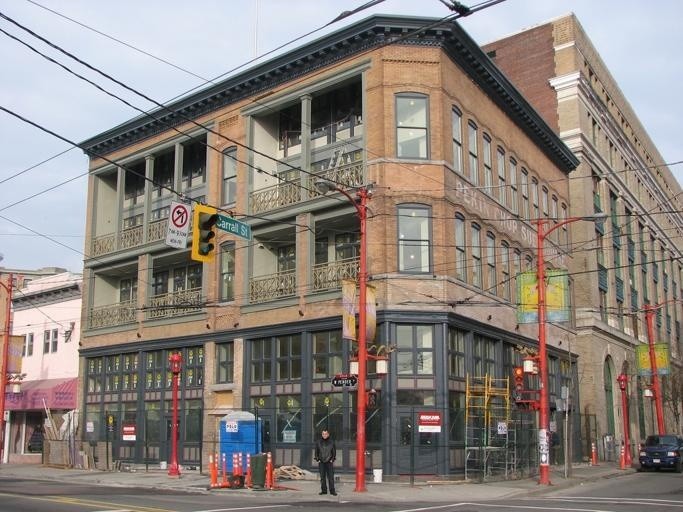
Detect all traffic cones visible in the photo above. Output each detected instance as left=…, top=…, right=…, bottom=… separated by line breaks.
left=208, top=450, right=275, bottom=491
left=620, top=445, right=627, bottom=470
left=588, top=440, right=597, bottom=465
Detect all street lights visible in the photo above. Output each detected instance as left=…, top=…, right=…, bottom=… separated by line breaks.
left=170, top=353, right=182, bottom=476
left=641, top=300, right=683, bottom=434
left=535, top=214, right=608, bottom=485
left=617, top=375, right=634, bottom=469
left=314, top=179, right=368, bottom=491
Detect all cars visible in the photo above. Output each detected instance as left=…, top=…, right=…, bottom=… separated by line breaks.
left=639, top=434, right=682, bottom=468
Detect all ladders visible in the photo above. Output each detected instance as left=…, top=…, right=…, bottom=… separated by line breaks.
left=465, top=372, right=516, bottom=483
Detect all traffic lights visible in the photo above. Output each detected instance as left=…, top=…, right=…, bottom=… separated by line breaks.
left=191, top=206, right=219, bottom=264
left=514, top=367, right=525, bottom=391
left=106, top=413, right=115, bottom=429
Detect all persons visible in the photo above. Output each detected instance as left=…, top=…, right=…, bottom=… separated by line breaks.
left=314, top=429, right=338, bottom=496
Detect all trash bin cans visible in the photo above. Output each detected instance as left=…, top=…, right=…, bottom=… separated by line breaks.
left=251, top=453, right=266, bottom=489
